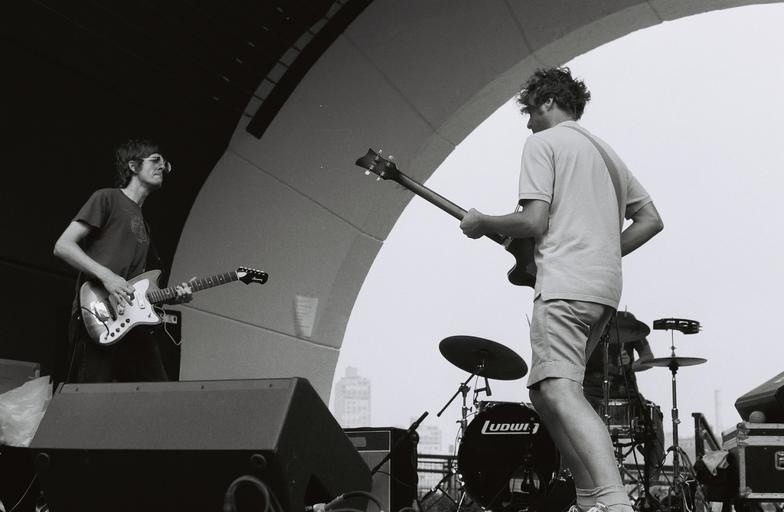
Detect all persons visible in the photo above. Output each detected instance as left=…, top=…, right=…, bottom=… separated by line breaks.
left=585, top=309, right=666, bottom=498
left=48, top=134, right=196, bottom=512
left=458, top=62, right=667, bottom=512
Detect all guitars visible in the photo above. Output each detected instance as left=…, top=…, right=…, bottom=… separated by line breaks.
left=354, top=148, right=537, bottom=290
left=80, top=266, right=269, bottom=346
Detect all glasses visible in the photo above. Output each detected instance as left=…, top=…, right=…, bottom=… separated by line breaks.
left=139, top=156, right=172, bottom=174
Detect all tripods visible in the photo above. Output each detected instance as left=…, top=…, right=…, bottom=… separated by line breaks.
left=639, top=369, right=717, bottom=512
left=419, top=387, right=483, bottom=511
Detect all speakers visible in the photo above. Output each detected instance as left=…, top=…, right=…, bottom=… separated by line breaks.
left=342, top=426, right=416, bottom=512
left=734, top=369, right=783, bottom=423
left=29, top=375, right=373, bottom=512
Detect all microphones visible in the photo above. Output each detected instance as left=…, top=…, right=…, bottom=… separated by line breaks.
left=520, top=455, right=534, bottom=492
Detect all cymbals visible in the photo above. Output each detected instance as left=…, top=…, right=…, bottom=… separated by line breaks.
left=640, top=357, right=706, bottom=368
left=603, top=314, right=650, bottom=344
left=440, top=337, right=528, bottom=380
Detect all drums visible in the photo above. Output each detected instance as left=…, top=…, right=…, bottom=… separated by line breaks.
left=589, top=399, right=662, bottom=439
left=455, top=400, right=577, bottom=509
